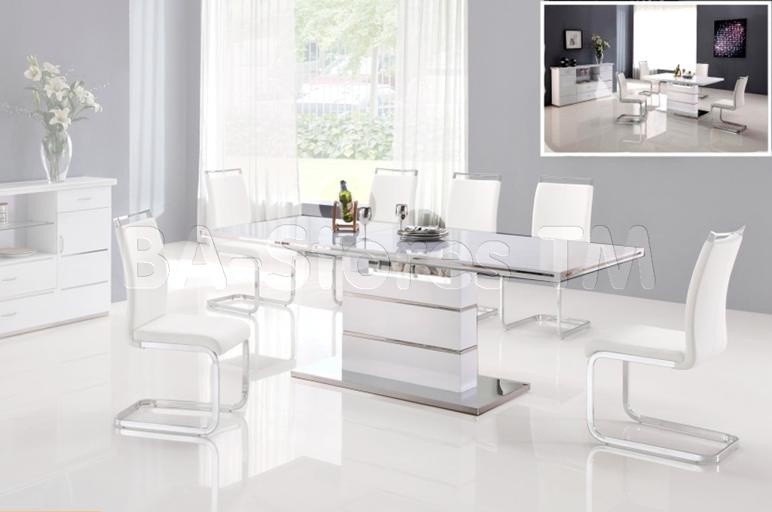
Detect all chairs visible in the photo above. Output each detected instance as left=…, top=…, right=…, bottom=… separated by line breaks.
left=113, top=209, right=250, bottom=437
left=586, top=226, right=749, bottom=463
left=200, top=166, right=296, bottom=315
left=365, top=168, right=418, bottom=222
left=446, top=173, right=501, bottom=233
left=497, top=182, right=594, bottom=339
left=617, top=60, right=749, bottom=135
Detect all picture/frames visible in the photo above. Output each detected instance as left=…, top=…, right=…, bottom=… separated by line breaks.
left=563, top=26, right=584, bottom=51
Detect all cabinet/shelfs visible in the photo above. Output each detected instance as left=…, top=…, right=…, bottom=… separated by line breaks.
left=1, top=176, right=120, bottom=341
left=549, top=62, right=615, bottom=108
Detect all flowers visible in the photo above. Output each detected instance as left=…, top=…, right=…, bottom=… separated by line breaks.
left=591, top=33, right=612, bottom=62
left=1, top=49, right=111, bottom=181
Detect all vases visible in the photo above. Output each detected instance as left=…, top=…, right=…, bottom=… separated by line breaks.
left=594, top=52, right=604, bottom=65
left=36, top=128, right=76, bottom=180
